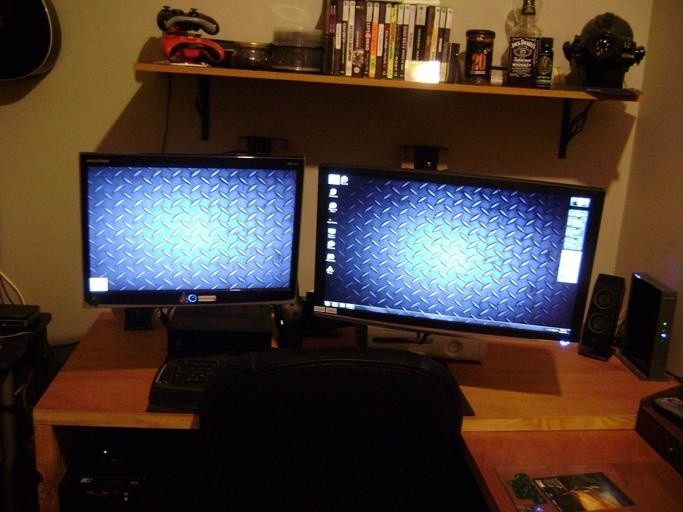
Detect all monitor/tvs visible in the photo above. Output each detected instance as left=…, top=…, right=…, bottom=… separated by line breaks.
left=312, top=166, right=605, bottom=363
left=81, top=153, right=306, bottom=350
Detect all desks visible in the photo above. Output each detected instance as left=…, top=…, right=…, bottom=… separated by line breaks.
left=31, top=312, right=683, bottom=511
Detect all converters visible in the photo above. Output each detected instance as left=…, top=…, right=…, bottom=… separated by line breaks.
left=279, top=321, right=302, bottom=350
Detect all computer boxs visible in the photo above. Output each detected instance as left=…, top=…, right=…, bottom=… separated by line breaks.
left=59, top=449, right=146, bottom=512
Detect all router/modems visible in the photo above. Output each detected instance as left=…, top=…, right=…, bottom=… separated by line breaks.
left=0, top=304, right=40, bottom=328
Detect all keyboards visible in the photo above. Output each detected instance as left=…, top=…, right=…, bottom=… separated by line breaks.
left=149, top=357, right=229, bottom=413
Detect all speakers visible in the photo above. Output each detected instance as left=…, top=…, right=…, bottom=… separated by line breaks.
left=578, top=273, right=626, bottom=362
left=123, top=308, right=154, bottom=330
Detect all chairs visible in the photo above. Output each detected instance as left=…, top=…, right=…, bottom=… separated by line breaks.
left=196, top=345, right=469, bottom=512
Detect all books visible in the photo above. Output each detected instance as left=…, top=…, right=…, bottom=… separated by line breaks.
left=323, top=1, right=460, bottom=84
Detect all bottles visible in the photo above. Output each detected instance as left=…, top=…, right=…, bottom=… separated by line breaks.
left=505, top=0, right=541, bottom=87
left=535, top=36, right=554, bottom=89
